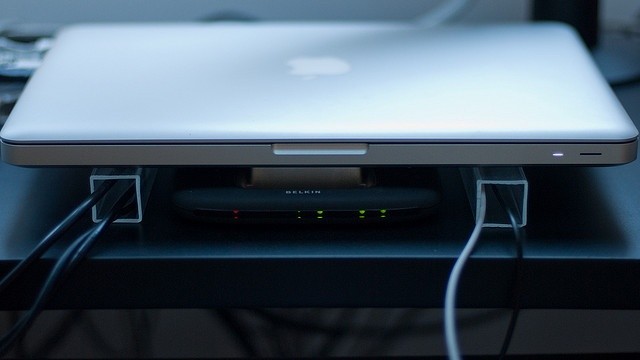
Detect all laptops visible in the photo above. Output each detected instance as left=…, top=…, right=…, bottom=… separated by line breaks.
left=1, top=19, right=639, bottom=168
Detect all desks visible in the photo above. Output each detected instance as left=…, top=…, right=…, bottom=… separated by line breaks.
left=1, top=30, right=640, bottom=359
